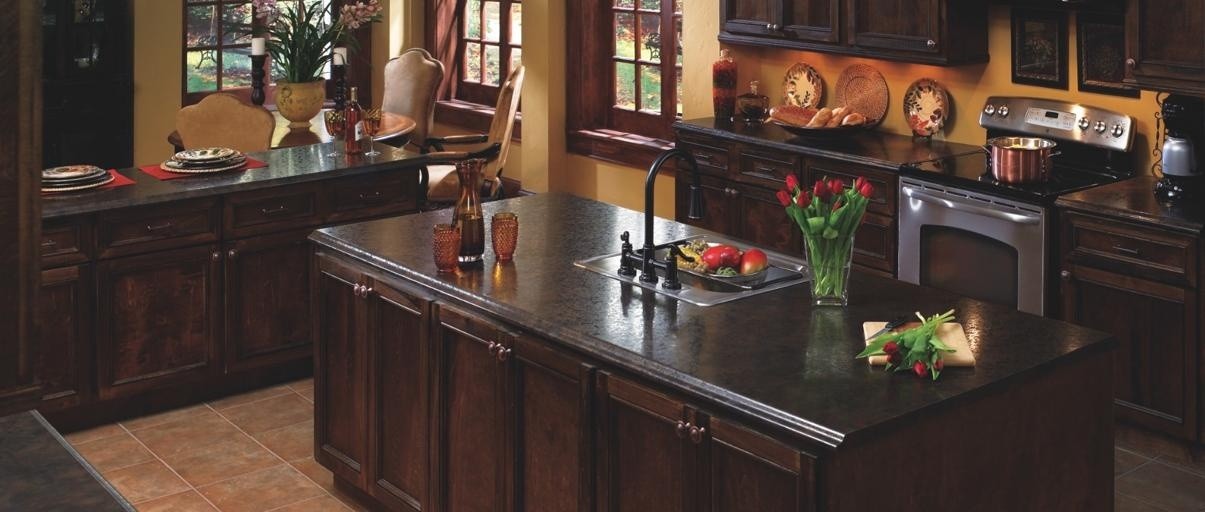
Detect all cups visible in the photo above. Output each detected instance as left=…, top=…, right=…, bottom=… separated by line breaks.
left=325, top=110, right=343, bottom=157
left=433, top=224, right=461, bottom=272
left=492, top=213, right=518, bottom=263
left=802, top=234, right=854, bottom=306
left=361, top=109, right=381, bottom=156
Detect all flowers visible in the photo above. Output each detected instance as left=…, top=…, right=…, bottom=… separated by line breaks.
left=776, top=175, right=873, bottom=297
left=223, top=0, right=383, bottom=83
left=854, top=308, right=957, bottom=382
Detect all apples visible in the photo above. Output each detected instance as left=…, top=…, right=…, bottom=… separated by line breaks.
left=739, top=249, right=768, bottom=275
left=700, top=244, right=743, bottom=269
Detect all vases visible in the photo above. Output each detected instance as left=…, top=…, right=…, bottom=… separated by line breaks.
left=274, top=77, right=327, bottom=127
left=802, top=234, right=854, bottom=308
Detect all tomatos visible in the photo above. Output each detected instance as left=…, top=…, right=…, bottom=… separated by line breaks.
left=741, top=248, right=768, bottom=275
left=701, top=244, right=741, bottom=270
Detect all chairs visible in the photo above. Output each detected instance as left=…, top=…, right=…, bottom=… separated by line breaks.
left=175, top=92, right=276, bottom=151
left=417, top=65, right=526, bottom=212
left=379, top=47, right=445, bottom=144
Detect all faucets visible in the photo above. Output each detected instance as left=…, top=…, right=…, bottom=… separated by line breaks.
left=618, top=149, right=705, bottom=290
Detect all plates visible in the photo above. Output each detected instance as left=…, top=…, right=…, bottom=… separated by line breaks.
left=159, top=160, right=246, bottom=173
left=773, top=119, right=861, bottom=139
left=834, top=64, right=888, bottom=126
left=783, top=63, right=822, bottom=109
left=707, top=265, right=771, bottom=287
left=41, top=175, right=115, bottom=192
left=903, top=78, right=950, bottom=137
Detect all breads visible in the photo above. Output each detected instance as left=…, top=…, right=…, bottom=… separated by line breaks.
left=768, top=104, right=821, bottom=127
left=824, top=105, right=851, bottom=129
left=804, top=107, right=832, bottom=129
left=842, top=110, right=866, bottom=126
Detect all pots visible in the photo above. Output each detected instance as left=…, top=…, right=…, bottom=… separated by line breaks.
left=982, top=136, right=1061, bottom=184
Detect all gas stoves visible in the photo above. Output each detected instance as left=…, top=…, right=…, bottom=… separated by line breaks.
left=897, top=96, right=1139, bottom=317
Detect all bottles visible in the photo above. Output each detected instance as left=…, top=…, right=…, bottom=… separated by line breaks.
left=344, top=86, right=363, bottom=156
left=713, top=49, right=738, bottom=120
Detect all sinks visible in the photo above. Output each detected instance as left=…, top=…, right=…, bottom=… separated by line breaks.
left=635, top=234, right=807, bottom=295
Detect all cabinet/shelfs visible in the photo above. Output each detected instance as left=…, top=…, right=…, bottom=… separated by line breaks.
left=95, top=197, right=225, bottom=409
left=843, top=0, right=990, bottom=69
left=716, top=1, right=843, bottom=55
left=330, top=166, right=420, bottom=225
left=312, top=244, right=434, bottom=512
left=223, top=180, right=331, bottom=383
left=675, top=128, right=801, bottom=259
left=593, top=328, right=1118, bottom=511
left=801, top=151, right=897, bottom=278
left=1053, top=209, right=1202, bottom=445
left=28, top=212, right=94, bottom=418
left=429, top=299, right=597, bottom=512
